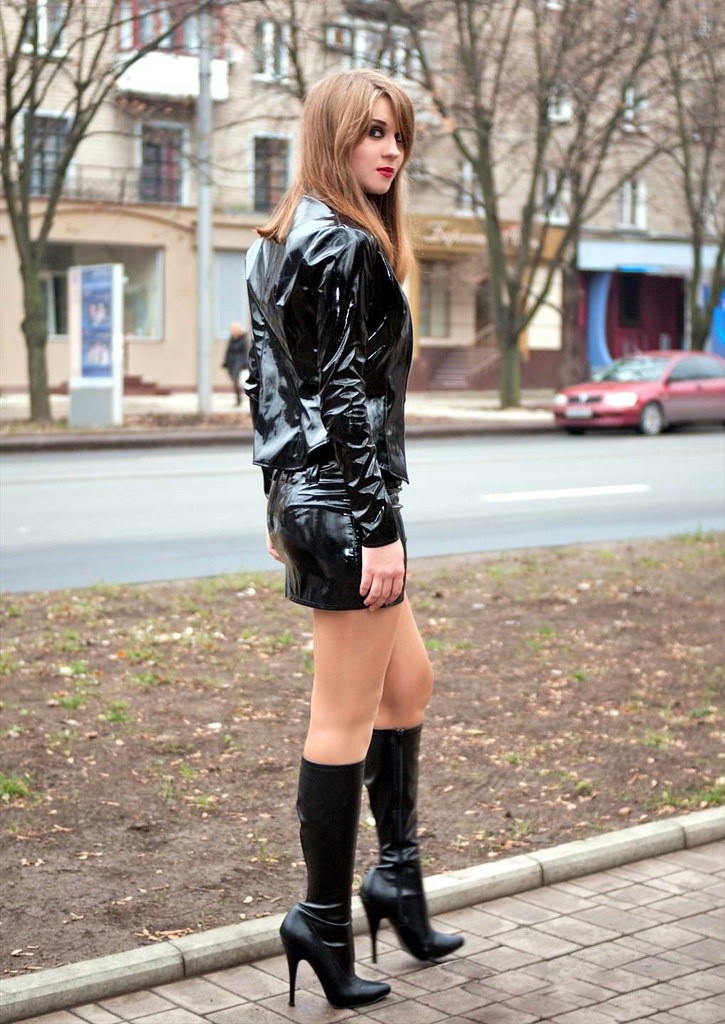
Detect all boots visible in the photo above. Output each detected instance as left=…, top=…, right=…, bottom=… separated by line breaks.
left=279, top=756, right=390, bottom=1008
left=363, top=724, right=464, bottom=961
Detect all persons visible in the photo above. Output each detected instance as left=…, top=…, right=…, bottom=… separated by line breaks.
left=222, top=323, right=249, bottom=406
left=244, top=67, right=466, bottom=1010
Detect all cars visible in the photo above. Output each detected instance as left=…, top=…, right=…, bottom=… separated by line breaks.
left=550, top=350, right=725, bottom=437
